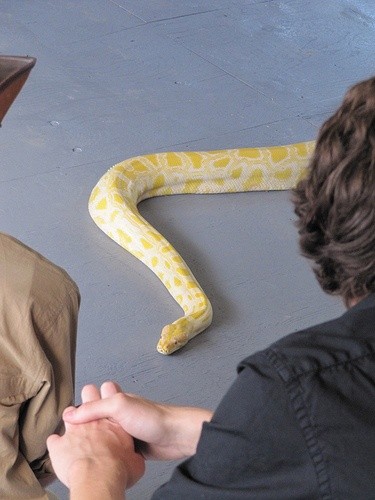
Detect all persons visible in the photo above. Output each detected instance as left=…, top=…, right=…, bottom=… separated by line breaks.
left=1, top=228, right=82, bottom=500
left=45, top=74, right=375, bottom=500
